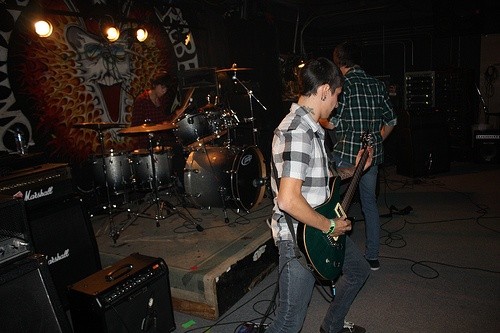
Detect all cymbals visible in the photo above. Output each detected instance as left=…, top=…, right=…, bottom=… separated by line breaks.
left=214, top=67, right=256, bottom=72
left=70, top=122, right=129, bottom=130
left=118, top=124, right=181, bottom=134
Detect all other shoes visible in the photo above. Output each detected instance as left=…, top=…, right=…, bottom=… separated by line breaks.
left=319, top=320, right=367, bottom=333
left=367, top=260, right=380, bottom=270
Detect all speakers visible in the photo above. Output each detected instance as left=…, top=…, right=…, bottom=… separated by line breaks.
left=397, top=106, right=475, bottom=178
left=0, top=150, right=176, bottom=333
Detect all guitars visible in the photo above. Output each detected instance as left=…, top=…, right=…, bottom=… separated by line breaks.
left=297, top=136, right=374, bottom=285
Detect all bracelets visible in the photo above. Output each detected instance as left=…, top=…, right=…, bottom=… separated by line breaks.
left=322, top=219, right=335, bottom=235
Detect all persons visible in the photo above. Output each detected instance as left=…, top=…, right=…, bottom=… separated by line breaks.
left=132, top=73, right=183, bottom=187
left=262, top=56, right=373, bottom=333
left=324, top=43, right=398, bottom=270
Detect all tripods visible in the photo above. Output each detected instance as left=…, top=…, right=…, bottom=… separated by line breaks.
left=88, top=120, right=242, bottom=244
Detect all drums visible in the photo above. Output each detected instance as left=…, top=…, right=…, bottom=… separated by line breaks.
left=88, top=103, right=268, bottom=213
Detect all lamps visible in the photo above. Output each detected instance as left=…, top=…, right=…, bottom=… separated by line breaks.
left=176, top=31, right=191, bottom=45
left=29, top=17, right=55, bottom=38
left=100, top=24, right=149, bottom=43
